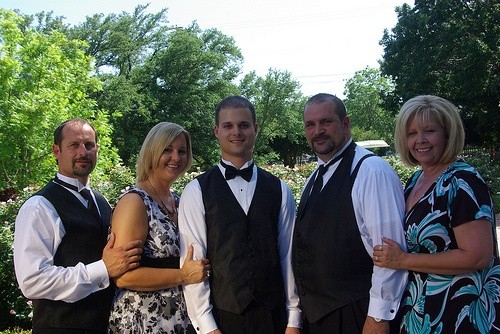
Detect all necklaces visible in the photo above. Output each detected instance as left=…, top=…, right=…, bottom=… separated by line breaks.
left=146, top=177, right=178, bottom=217
left=411, top=171, right=438, bottom=197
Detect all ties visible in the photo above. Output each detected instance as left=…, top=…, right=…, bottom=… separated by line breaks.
left=54, top=176, right=100, bottom=225
left=300, top=147, right=348, bottom=221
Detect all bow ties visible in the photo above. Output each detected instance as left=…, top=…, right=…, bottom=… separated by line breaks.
left=220, top=160, right=254, bottom=182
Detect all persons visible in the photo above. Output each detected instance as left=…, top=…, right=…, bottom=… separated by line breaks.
left=291, top=93, right=408, bottom=334
left=178, top=96, right=304, bottom=334
left=373, top=94, right=500, bottom=334
left=14, top=118, right=143, bottom=334
left=109, top=122, right=212, bottom=334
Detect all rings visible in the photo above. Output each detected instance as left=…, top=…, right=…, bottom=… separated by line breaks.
left=375, top=256, right=379, bottom=262
left=378, top=245, right=383, bottom=252
left=206, top=270, right=211, bottom=277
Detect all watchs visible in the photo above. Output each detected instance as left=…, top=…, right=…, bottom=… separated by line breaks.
left=371, top=316, right=385, bottom=323
